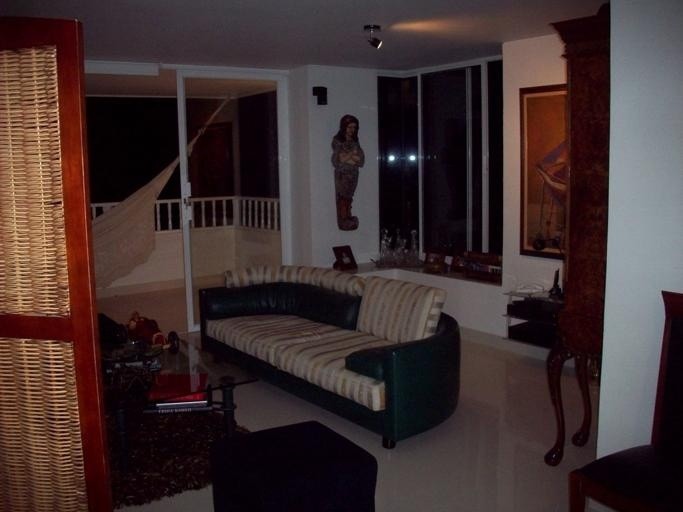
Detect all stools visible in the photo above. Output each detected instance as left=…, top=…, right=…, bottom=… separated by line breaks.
left=206, top=419, right=377, bottom=511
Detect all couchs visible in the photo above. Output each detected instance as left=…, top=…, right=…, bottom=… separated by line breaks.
left=197, top=265, right=461, bottom=451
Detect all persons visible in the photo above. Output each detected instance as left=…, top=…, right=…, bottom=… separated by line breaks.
left=330, top=113, right=366, bottom=231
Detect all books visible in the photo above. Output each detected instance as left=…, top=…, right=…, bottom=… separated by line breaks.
left=143, top=392, right=213, bottom=414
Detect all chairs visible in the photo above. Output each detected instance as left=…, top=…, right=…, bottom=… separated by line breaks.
left=565, top=291, right=682, bottom=512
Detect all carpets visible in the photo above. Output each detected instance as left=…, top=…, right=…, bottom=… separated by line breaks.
left=105, top=410, right=251, bottom=507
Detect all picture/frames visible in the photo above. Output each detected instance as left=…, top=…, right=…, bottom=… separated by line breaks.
left=517, top=84, right=568, bottom=259
left=332, top=245, right=357, bottom=272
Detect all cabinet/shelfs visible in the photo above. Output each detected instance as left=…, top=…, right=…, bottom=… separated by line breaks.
left=500, top=290, right=571, bottom=358
